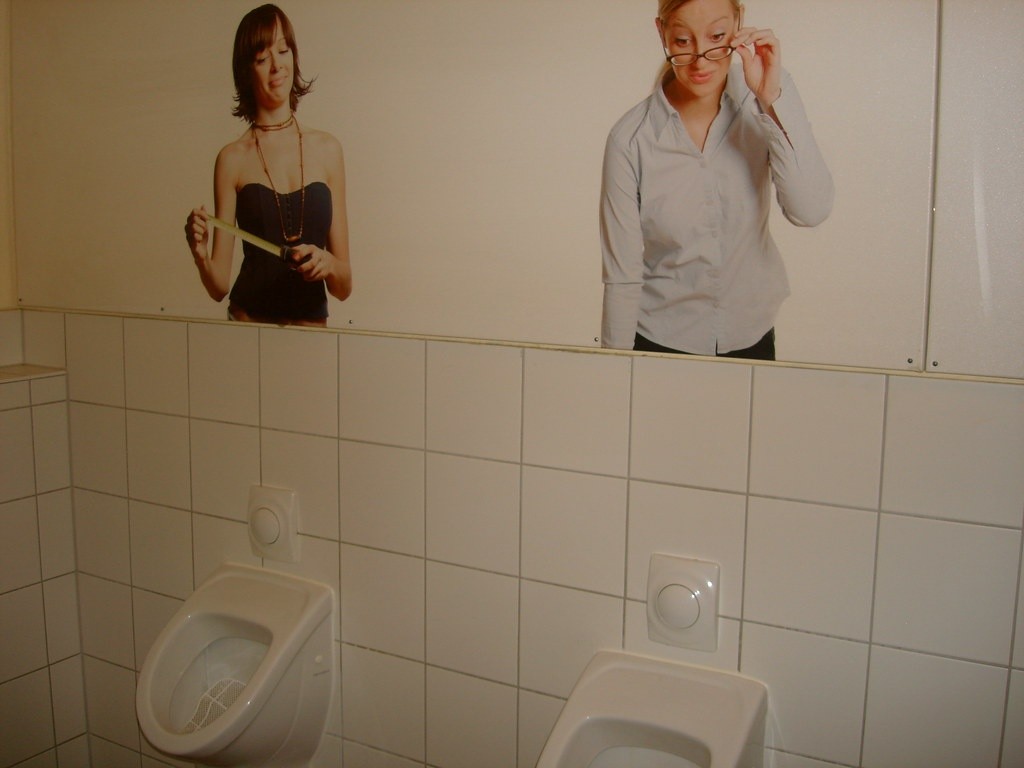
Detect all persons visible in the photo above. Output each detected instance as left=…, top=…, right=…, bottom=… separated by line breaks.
left=600, top=0, right=833, bottom=360
left=184, top=5, right=353, bottom=328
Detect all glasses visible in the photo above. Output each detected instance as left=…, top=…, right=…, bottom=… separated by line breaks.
left=661, top=8, right=740, bottom=66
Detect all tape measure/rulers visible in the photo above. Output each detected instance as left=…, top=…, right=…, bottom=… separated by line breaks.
left=205, top=214, right=311, bottom=266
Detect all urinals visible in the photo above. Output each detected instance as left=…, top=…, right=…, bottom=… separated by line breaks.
left=134, top=565, right=334, bottom=767
left=532, top=651, right=768, bottom=768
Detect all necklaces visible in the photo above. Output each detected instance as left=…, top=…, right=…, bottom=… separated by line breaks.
left=254, top=113, right=306, bottom=242
